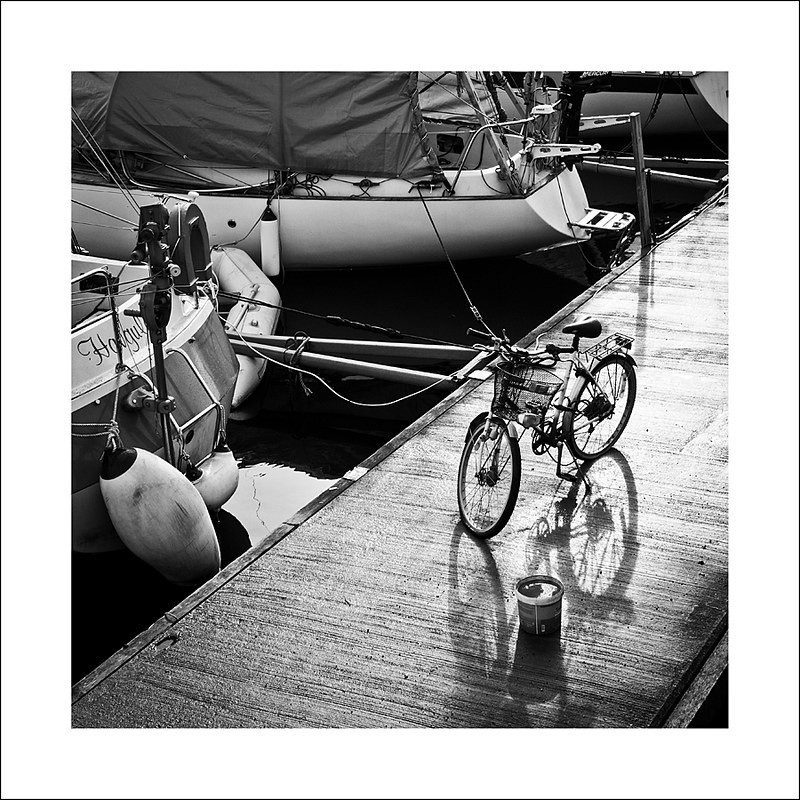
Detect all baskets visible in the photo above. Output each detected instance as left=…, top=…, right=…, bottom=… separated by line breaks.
left=492, top=361, right=564, bottom=421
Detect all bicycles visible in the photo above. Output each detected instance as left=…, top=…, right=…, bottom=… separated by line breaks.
left=457, top=314, right=639, bottom=541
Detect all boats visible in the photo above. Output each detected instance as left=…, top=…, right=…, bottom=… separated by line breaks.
left=71, top=202, right=241, bottom=555
left=488, top=71, right=729, bottom=162
left=72, top=71, right=600, bottom=266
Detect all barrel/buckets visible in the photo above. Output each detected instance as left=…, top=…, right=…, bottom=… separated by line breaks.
left=514, top=574, right=565, bottom=635
